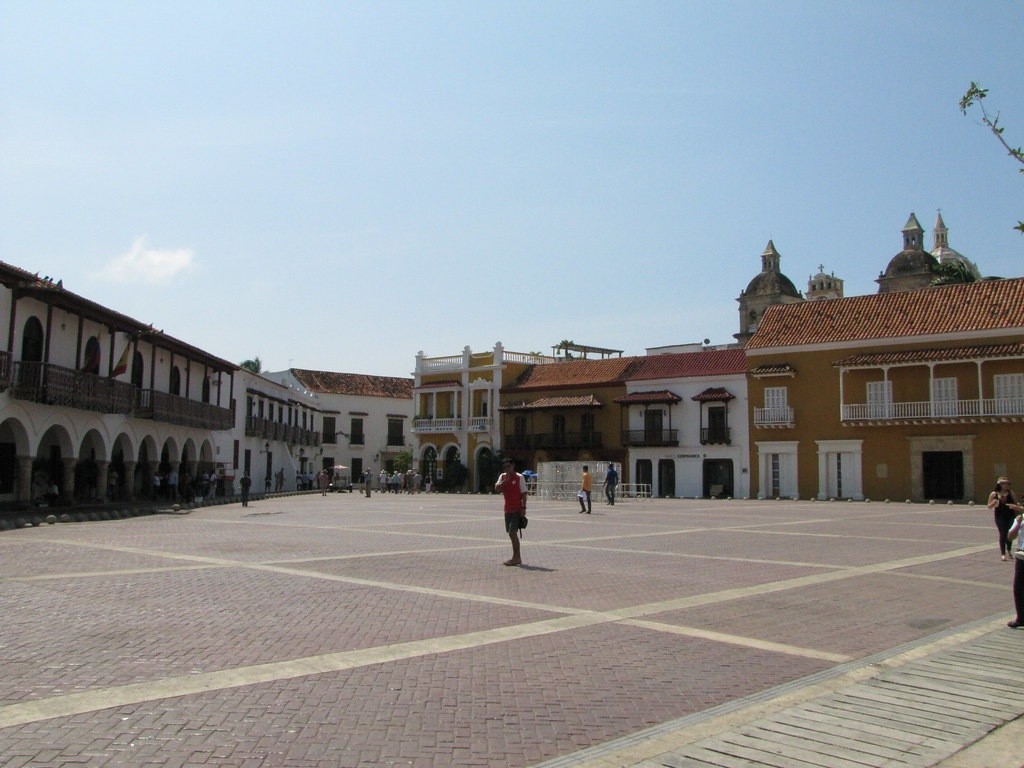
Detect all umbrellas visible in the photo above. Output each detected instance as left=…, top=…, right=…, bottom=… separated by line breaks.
left=329, top=464, right=350, bottom=469
left=522, top=470, right=537, bottom=490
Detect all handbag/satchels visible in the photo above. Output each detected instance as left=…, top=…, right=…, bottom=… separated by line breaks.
left=518, top=517, right=528, bottom=529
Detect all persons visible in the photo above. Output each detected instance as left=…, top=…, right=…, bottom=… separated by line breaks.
left=495, top=458, right=528, bottom=566
left=87, top=470, right=125, bottom=502
left=987, top=476, right=1017, bottom=561
left=264, top=474, right=271, bottom=494
left=379, top=469, right=422, bottom=495
left=319, top=469, right=330, bottom=496
left=602, top=464, right=618, bottom=505
left=359, top=468, right=373, bottom=497
left=275, top=468, right=286, bottom=492
left=238, top=471, right=251, bottom=507
left=579, top=465, right=592, bottom=514
left=32, top=467, right=59, bottom=508
left=296, top=471, right=321, bottom=491
left=153, top=467, right=178, bottom=501
left=1006, top=495, right=1024, bottom=628
left=424, top=473, right=432, bottom=494
left=180, top=469, right=218, bottom=504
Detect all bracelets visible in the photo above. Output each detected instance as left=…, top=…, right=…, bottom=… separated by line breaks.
left=522, top=506, right=526, bottom=509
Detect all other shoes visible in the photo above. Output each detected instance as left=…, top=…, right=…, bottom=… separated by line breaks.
left=1007, top=618, right=1024, bottom=627
left=585, top=511, right=591, bottom=514
left=503, top=559, right=522, bottom=566
left=1008, top=551, right=1013, bottom=559
left=580, top=509, right=586, bottom=513
left=606, top=501, right=614, bottom=505
left=1001, top=555, right=1006, bottom=561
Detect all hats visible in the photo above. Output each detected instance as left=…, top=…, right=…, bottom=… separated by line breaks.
left=996, top=476, right=1010, bottom=483
left=394, top=471, right=399, bottom=474
left=381, top=470, right=385, bottom=473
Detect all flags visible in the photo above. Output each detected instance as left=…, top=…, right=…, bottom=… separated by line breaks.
left=83, top=335, right=130, bottom=377
